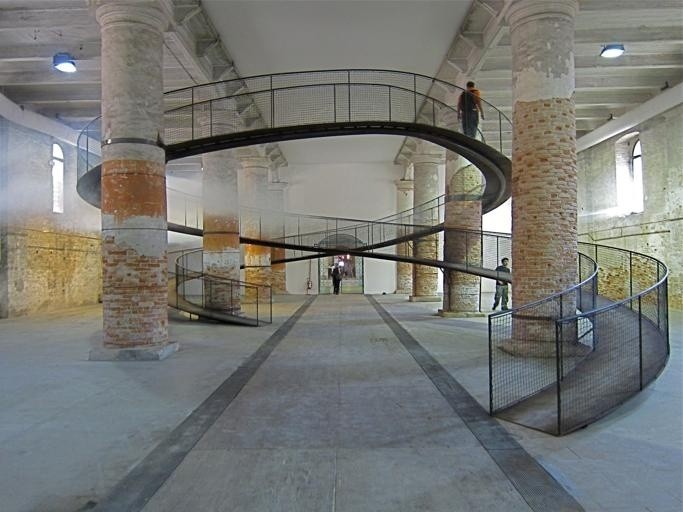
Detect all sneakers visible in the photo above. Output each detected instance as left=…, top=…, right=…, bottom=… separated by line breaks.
left=492, top=306, right=509, bottom=310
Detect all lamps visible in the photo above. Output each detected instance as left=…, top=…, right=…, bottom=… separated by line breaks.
left=600, top=44, right=624, bottom=59
left=53, top=53, right=76, bottom=73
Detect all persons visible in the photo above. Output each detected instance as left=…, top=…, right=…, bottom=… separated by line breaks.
left=456, top=80, right=484, bottom=139
left=491, top=256, right=512, bottom=311
left=329, top=266, right=342, bottom=293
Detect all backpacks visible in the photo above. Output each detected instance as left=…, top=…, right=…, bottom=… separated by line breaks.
left=460, top=90, right=478, bottom=113
